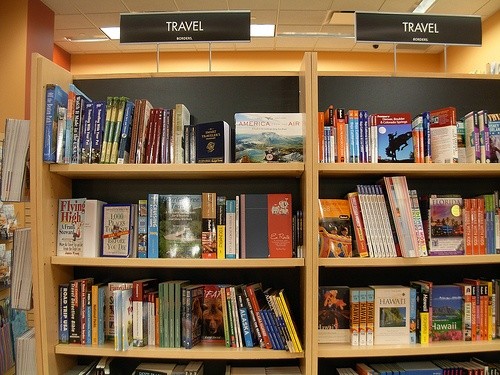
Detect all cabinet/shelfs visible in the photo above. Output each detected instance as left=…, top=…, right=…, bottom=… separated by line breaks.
left=30, top=51, right=500, bottom=375
